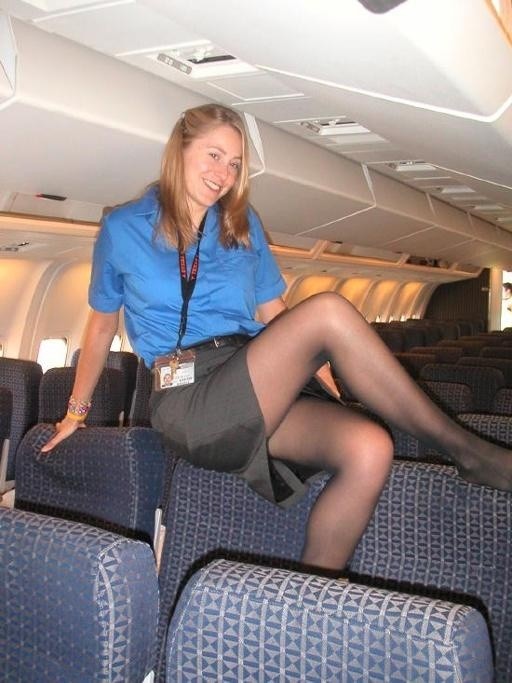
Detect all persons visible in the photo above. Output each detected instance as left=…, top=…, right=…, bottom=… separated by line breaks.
left=503, top=282, right=512, bottom=313
left=40, top=103, right=512, bottom=578
left=162, top=374, right=173, bottom=387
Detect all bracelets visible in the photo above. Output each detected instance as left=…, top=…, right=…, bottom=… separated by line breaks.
left=67, top=395, right=91, bottom=422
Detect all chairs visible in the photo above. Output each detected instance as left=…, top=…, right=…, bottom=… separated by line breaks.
left=2, top=319, right=511, bottom=682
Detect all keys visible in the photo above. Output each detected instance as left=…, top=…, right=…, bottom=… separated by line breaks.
left=169, top=360, right=179, bottom=380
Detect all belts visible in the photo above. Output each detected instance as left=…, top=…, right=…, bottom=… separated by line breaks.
left=180, top=333, right=251, bottom=355
